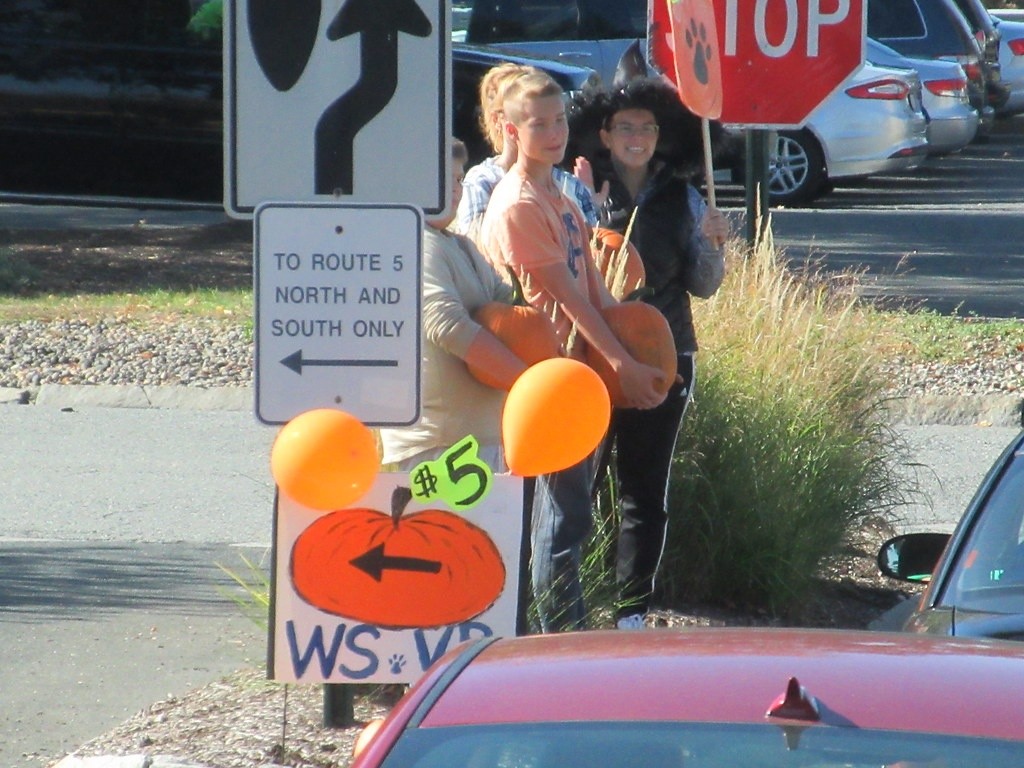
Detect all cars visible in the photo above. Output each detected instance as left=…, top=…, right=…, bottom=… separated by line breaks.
left=348, top=626, right=1023, bottom=768
left=451, top=0, right=930, bottom=202
left=908, top=55, right=978, bottom=161
left=957, top=0, right=1024, bottom=115
left=450, top=45, right=600, bottom=175
left=876, top=420, right=1023, bottom=640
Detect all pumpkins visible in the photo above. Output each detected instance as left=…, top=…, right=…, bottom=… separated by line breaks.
left=585, top=302, right=677, bottom=408
left=586, top=226, right=647, bottom=303
left=465, top=263, right=559, bottom=392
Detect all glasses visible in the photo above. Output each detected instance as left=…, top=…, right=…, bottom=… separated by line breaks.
left=609, top=122, right=659, bottom=135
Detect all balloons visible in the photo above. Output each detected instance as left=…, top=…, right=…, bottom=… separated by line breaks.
left=270, top=407, right=380, bottom=513
left=502, top=358, right=612, bottom=476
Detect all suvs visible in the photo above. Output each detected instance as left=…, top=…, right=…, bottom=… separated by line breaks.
left=869, top=1, right=994, bottom=141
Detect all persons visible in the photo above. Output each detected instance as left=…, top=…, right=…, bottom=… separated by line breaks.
left=441, top=65, right=597, bottom=235
left=379, top=135, right=568, bottom=635
left=570, top=76, right=725, bottom=632
left=476, top=73, right=682, bottom=634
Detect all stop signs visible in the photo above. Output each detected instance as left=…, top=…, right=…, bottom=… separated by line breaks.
left=643, top=0, right=868, bottom=131
